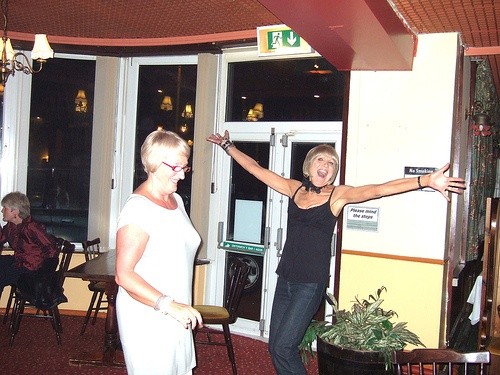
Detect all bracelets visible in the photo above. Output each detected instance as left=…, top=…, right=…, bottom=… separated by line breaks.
left=221, top=141, right=232, bottom=150
left=418, top=176, right=425, bottom=189
left=154, top=295, right=174, bottom=315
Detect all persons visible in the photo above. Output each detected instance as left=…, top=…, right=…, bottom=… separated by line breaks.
left=115, top=130, right=204, bottom=375
left=0, top=192, right=58, bottom=298
left=207, top=130, right=465, bottom=375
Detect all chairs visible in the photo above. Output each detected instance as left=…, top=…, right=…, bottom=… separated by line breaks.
left=191, top=261, right=252, bottom=375
left=3, top=233, right=75, bottom=347
left=80, top=237, right=106, bottom=336
left=391, top=347, right=491, bottom=375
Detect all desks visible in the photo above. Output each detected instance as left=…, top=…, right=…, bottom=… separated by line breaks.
left=63, top=248, right=127, bottom=368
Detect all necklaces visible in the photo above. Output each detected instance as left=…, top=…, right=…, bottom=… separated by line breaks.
left=303, top=177, right=328, bottom=194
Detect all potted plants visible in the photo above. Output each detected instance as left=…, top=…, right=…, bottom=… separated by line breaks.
left=298, top=286, right=428, bottom=375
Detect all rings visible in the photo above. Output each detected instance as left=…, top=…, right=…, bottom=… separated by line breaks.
left=186, top=319, right=190, bottom=323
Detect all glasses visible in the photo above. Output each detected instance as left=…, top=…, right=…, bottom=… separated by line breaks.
left=162, top=161, right=192, bottom=173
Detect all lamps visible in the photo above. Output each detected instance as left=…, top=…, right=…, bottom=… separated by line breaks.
left=0, top=0, right=55, bottom=88
left=464, top=101, right=492, bottom=136
